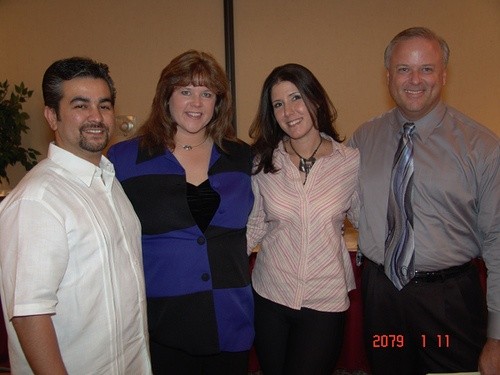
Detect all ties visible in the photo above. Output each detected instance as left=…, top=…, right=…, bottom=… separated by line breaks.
left=385, top=123, right=416, bottom=292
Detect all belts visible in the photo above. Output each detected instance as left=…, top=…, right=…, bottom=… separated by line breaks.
left=377, top=260, right=472, bottom=282
